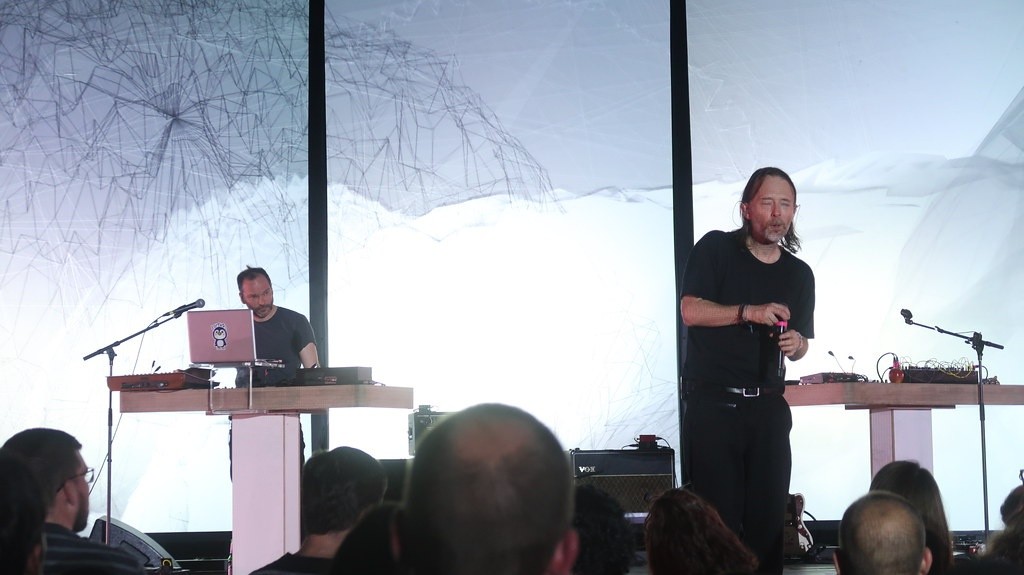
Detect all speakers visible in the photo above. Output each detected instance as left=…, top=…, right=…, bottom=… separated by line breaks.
left=408, top=411, right=451, bottom=457
left=566, top=448, right=675, bottom=524
left=87, top=516, right=181, bottom=569
left=376, top=458, right=408, bottom=503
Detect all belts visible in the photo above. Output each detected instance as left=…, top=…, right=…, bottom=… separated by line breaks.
left=690, top=384, right=781, bottom=397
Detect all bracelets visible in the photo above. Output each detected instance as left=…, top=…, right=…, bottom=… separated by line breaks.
left=796, top=331, right=803, bottom=351
left=737, top=304, right=746, bottom=322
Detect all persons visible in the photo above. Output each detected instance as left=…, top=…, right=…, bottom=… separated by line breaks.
left=400, top=402, right=578, bottom=575
left=1000, top=469, right=1024, bottom=531
left=573, top=483, right=634, bottom=575
left=679, top=167, right=815, bottom=575
left=229, top=265, right=320, bottom=483
left=832, top=491, right=932, bottom=575
left=0, top=428, right=145, bottom=575
left=331, top=507, right=399, bottom=575
left=868, top=461, right=955, bottom=575
left=642, top=482, right=757, bottom=575
left=248, top=448, right=398, bottom=575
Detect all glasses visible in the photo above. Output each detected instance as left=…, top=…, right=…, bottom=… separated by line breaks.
left=56, top=466, right=95, bottom=494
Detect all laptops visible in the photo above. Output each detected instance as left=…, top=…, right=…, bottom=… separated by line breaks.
left=187, top=310, right=282, bottom=363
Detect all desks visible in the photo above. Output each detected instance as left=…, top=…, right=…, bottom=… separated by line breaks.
left=118, top=386, right=413, bottom=575
left=783, top=381, right=1024, bottom=484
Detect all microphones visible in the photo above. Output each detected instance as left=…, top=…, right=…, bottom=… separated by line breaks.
left=164, top=299, right=206, bottom=316
left=775, top=315, right=788, bottom=379
